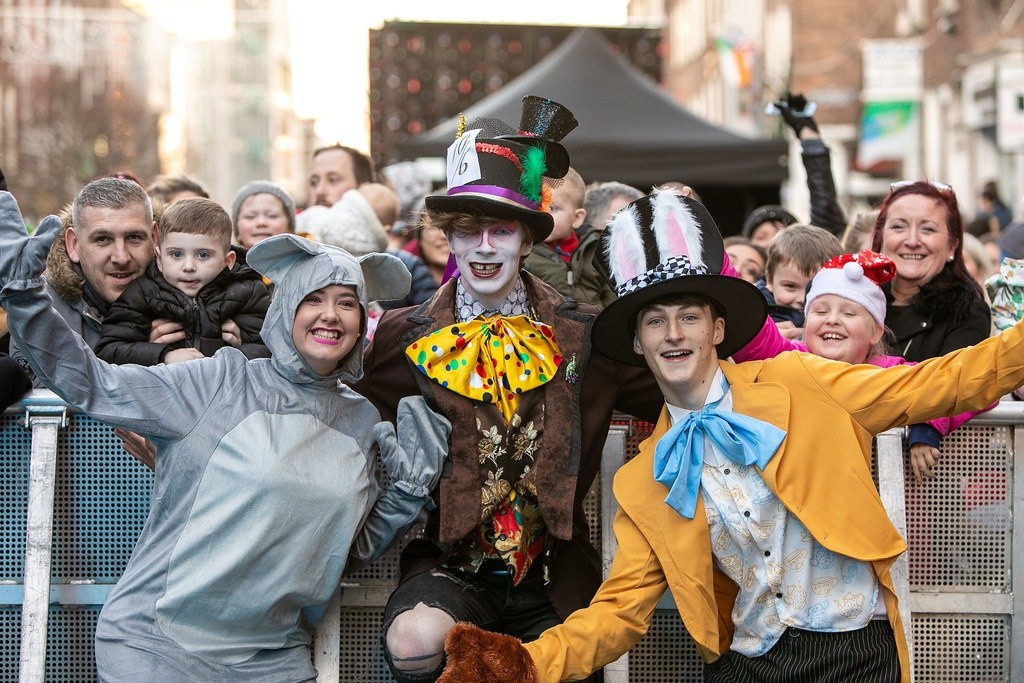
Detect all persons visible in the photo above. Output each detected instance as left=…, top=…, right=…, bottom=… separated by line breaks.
left=0, top=95, right=1024, bottom=683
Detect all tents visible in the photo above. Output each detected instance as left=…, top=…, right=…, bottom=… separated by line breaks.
left=398, top=29, right=790, bottom=189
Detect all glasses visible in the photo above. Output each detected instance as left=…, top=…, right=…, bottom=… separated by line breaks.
left=888, top=179, right=955, bottom=200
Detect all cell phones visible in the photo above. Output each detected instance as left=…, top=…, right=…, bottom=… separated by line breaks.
left=887, top=308, right=932, bottom=342
left=765, top=101, right=817, bottom=117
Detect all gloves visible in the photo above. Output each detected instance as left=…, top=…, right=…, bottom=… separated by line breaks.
left=773, top=91, right=824, bottom=137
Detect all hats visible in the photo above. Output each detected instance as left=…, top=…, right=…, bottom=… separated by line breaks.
left=232, top=182, right=296, bottom=236
left=591, top=187, right=769, bottom=366
left=423, top=139, right=555, bottom=246
left=804, top=250, right=895, bottom=328
